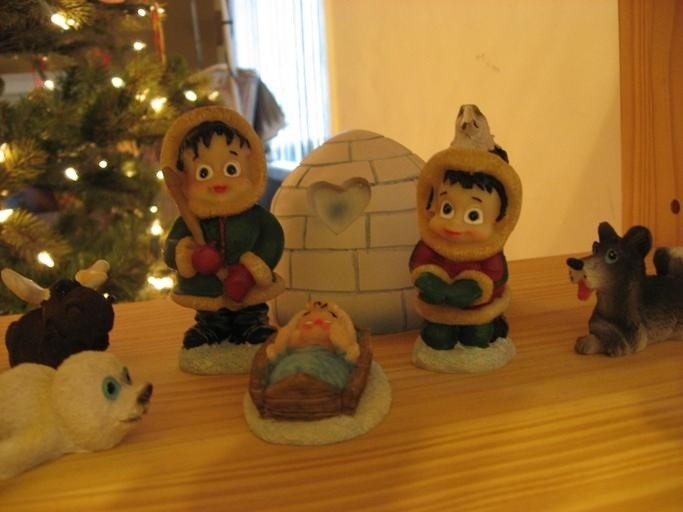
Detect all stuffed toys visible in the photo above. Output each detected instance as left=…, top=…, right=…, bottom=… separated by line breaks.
left=6, top=278, right=114, bottom=370
left=567, top=220, right=683, bottom=356
left=0, top=350, right=154, bottom=482
left=246, top=299, right=373, bottom=420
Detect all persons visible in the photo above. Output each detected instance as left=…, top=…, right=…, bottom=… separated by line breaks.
left=156, top=105, right=281, bottom=372
left=403, top=103, right=524, bottom=374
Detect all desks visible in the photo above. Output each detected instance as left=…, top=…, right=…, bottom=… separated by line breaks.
left=0, top=250, right=682, bottom=512
